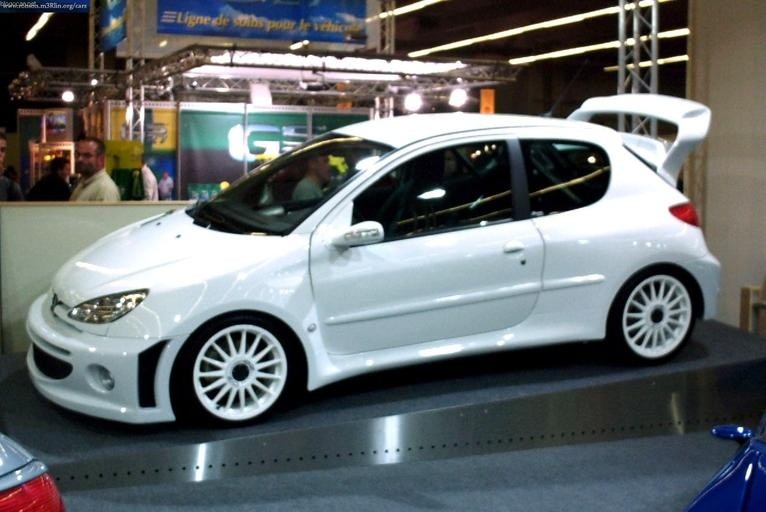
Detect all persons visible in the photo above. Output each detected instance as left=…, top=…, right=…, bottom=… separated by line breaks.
left=0, top=132, right=24, bottom=200
left=267, top=153, right=357, bottom=202
left=24, top=156, right=71, bottom=201
left=68, top=134, right=120, bottom=201
left=157, top=171, right=174, bottom=200
left=4, top=165, right=19, bottom=183
left=142, top=160, right=159, bottom=201
